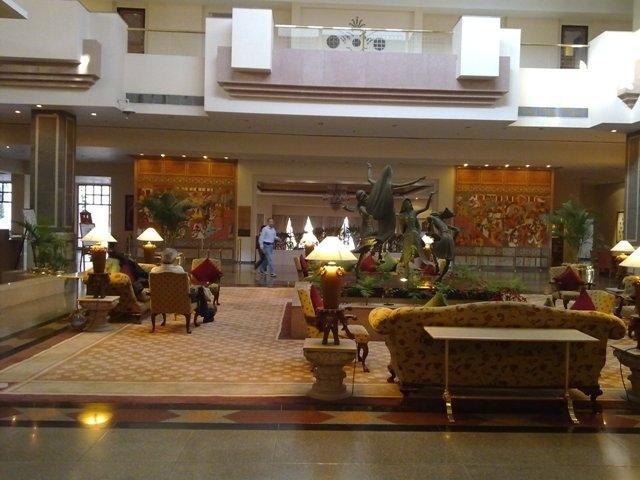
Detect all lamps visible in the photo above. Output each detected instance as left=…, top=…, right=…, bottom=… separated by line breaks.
left=304, top=236, right=360, bottom=309
left=81, top=226, right=117, bottom=273
left=136, top=227, right=164, bottom=256
left=610, top=240, right=640, bottom=292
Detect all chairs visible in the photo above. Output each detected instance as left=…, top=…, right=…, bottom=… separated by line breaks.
left=296, top=288, right=371, bottom=373
left=75, top=256, right=222, bottom=334
left=547, top=265, right=614, bottom=309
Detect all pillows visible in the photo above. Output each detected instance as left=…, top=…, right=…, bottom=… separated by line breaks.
left=552, top=266, right=585, bottom=291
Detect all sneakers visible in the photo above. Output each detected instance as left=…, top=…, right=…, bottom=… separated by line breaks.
left=270, top=273, right=277, bottom=277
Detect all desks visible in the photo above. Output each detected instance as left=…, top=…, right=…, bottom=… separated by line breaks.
left=420, top=324, right=600, bottom=428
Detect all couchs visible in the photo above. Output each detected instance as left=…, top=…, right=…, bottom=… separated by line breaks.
left=368, top=299, right=626, bottom=418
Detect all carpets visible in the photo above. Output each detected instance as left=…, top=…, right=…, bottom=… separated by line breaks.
left=0, top=285, right=638, bottom=401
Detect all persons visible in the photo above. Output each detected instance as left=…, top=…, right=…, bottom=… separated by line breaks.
left=364, top=161, right=428, bottom=237
left=422, top=210, right=461, bottom=283
left=355, top=189, right=371, bottom=229
left=259, top=217, right=282, bottom=277
left=253, top=225, right=268, bottom=271
left=397, top=190, right=438, bottom=235
